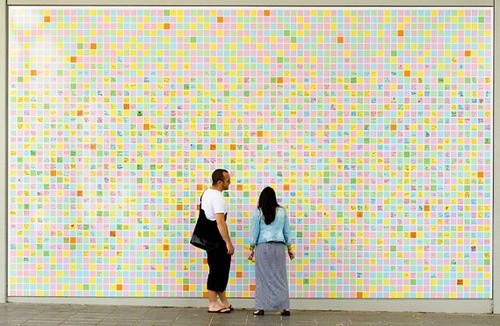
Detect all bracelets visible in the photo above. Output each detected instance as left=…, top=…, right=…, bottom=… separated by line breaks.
left=288, top=248, right=292, bottom=250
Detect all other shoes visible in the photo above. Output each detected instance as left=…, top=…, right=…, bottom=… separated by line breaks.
left=282, top=311, right=290, bottom=316
left=253, top=310, right=264, bottom=316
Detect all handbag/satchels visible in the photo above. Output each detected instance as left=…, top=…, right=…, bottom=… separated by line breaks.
left=190, top=209, right=227, bottom=251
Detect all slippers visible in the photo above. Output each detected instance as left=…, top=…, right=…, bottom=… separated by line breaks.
left=229, top=305, right=234, bottom=311
left=208, top=308, right=231, bottom=313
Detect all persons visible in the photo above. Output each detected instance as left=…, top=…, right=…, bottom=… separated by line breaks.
left=248, top=185, right=295, bottom=316
left=189, top=168, right=234, bottom=314
